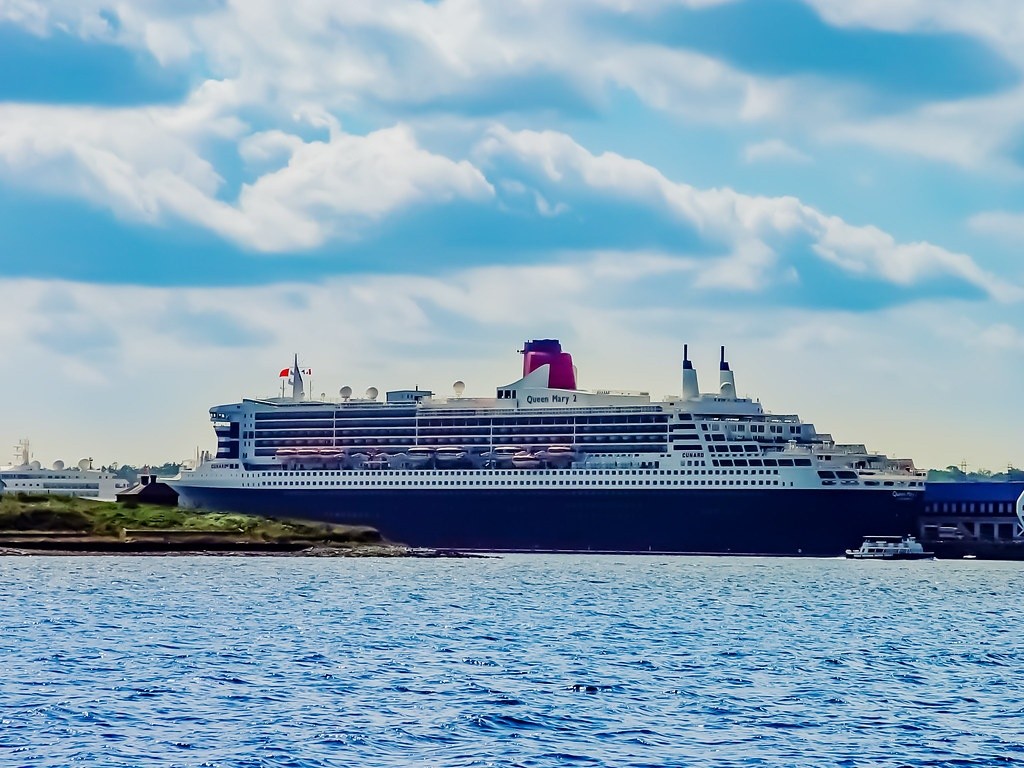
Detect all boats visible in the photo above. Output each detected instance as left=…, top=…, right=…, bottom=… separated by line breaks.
left=845, top=534, right=934, bottom=560
left=435, top=447, right=465, bottom=460
left=547, top=446, right=577, bottom=458
left=276, top=449, right=298, bottom=459
left=481, top=447, right=522, bottom=461
left=405, top=448, right=435, bottom=461
left=512, top=455, right=540, bottom=468
left=0, top=436, right=130, bottom=498
left=296, top=450, right=322, bottom=458
left=319, top=449, right=346, bottom=459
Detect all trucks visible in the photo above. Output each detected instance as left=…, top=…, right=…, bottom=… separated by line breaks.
left=936, top=527, right=966, bottom=541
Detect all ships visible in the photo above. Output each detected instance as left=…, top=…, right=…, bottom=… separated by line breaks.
left=155, top=338, right=929, bottom=558
